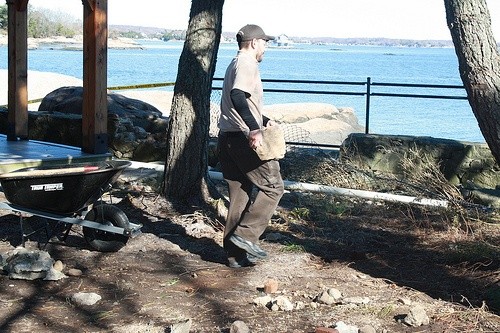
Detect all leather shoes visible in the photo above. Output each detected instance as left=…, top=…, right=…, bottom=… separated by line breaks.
left=227, top=255, right=256, bottom=268
left=230, top=234, right=269, bottom=258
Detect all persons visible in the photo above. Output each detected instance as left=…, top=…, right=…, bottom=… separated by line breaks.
left=218, top=24, right=284, bottom=268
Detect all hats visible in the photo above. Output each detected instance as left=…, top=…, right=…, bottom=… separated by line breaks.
left=236, top=24, right=275, bottom=42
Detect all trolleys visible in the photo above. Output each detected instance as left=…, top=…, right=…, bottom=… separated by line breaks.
left=0, top=158, right=130, bottom=253
